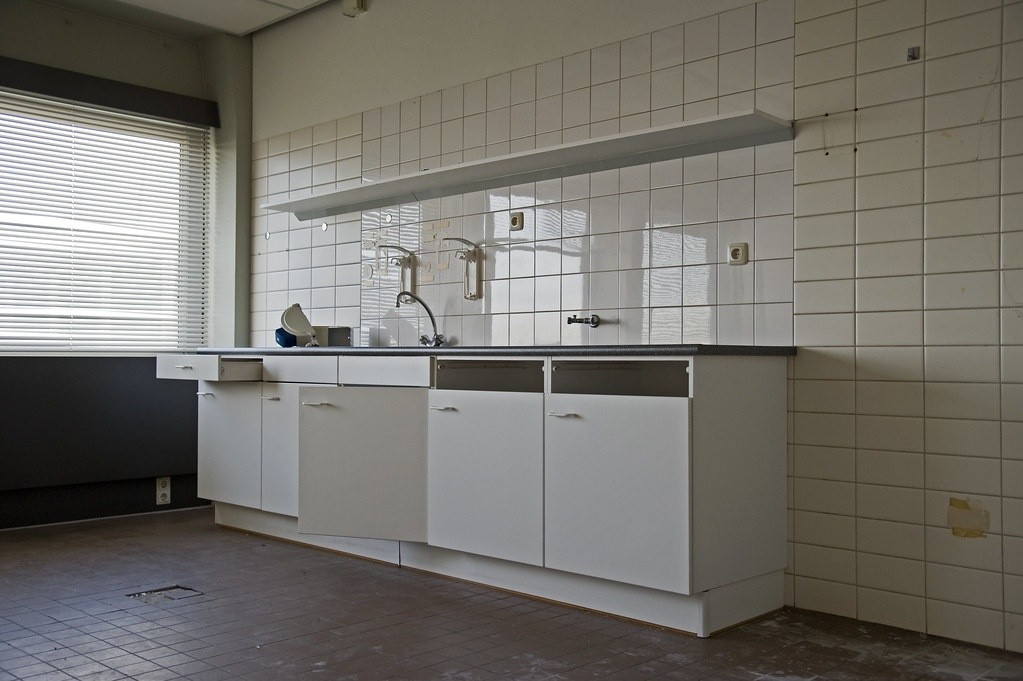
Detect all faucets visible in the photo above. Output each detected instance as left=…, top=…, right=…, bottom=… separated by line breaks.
left=396, top=291, right=444, bottom=347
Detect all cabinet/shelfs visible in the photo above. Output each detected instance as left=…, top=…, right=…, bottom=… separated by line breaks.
left=436, top=357, right=791, bottom=639
left=156, top=353, right=338, bottom=552
left=339, top=354, right=437, bottom=574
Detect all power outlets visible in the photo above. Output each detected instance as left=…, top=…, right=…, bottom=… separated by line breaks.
left=155, top=477, right=171, bottom=505
left=509, top=211, right=524, bottom=231
left=727, top=242, right=748, bottom=265
